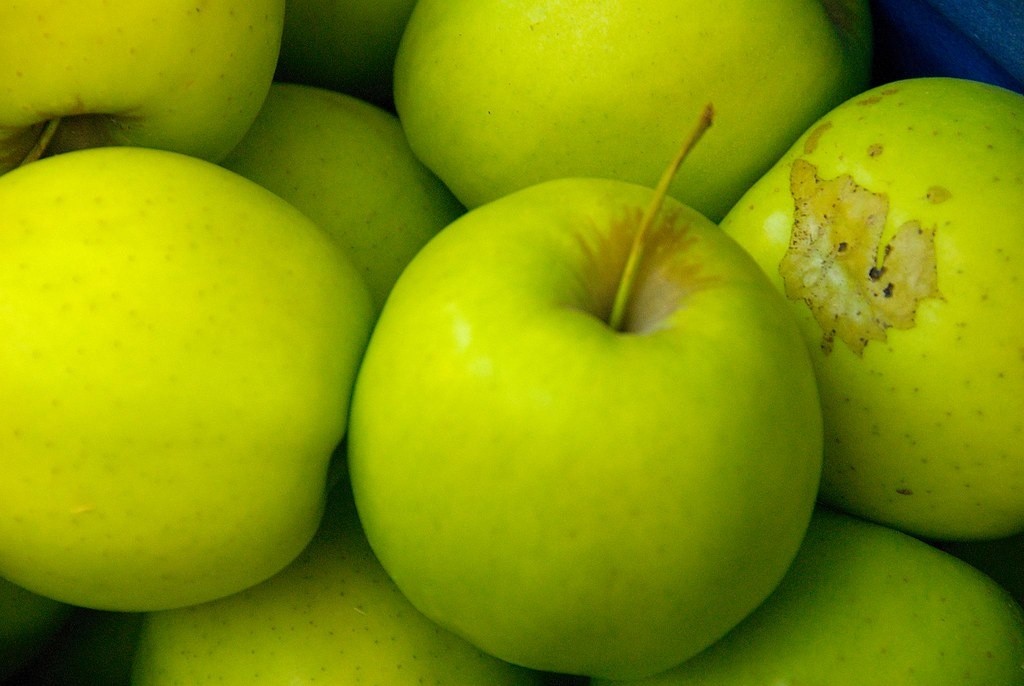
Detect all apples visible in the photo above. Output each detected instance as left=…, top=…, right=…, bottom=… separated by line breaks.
left=0, top=0, right=1024, bottom=686
